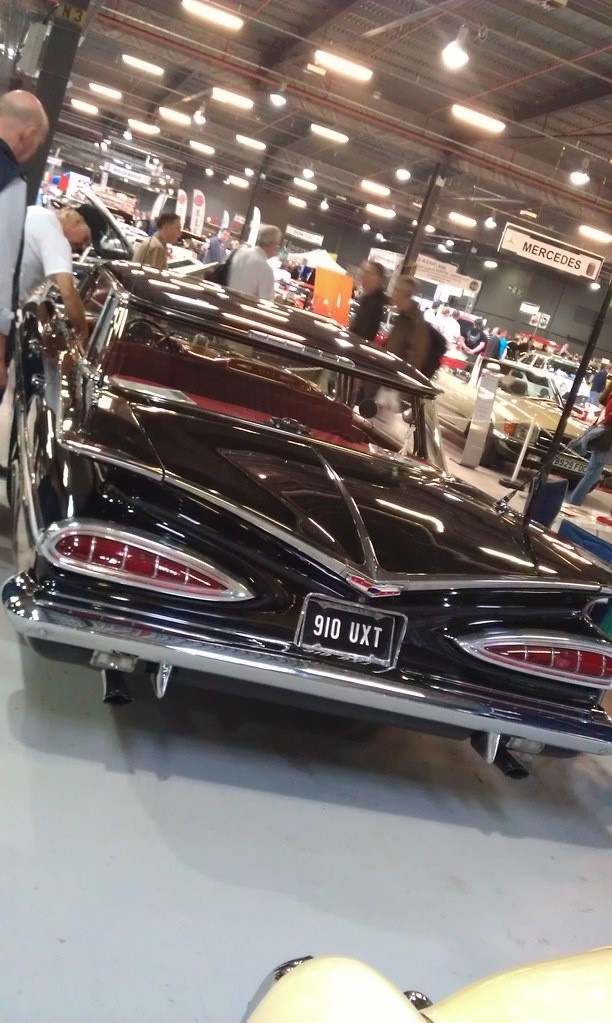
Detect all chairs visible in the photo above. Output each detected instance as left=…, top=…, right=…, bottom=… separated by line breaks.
left=510, top=381, right=529, bottom=395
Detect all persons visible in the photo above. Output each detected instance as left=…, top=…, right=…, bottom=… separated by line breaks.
left=227, top=226, right=282, bottom=301
left=18, top=203, right=107, bottom=342
left=517, top=337, right=535, bottom=354
left=366, top=275, right=429, bottom=410
left=589, top=363, right=607, bottom=406
left=424, top=302, right=519, bottom=362
left=202, top=229, right=230, bottom=265
left=133, top=214, right=181, bottom=269
left=350, top=260, right=385, bottom=341
left=541, top=344, right=547, bottom=352
left=571, top=380, right=612, bottom=507
left=560, top=344, right=583, bottom=363
left=0, top=91, right=49, bottom=405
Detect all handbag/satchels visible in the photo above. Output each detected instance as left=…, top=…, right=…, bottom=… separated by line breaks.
left=421, top=324, right=447, bottom=380
left=205, top=249, right=238, bottom=287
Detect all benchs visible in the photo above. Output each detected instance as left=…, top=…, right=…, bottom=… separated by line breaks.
left=99, top=342, right=372, bottom=443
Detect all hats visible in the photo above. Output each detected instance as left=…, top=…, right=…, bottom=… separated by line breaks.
left=75, top=204, right=107, bottom=256
left=475, top=319, right=482, bottom=328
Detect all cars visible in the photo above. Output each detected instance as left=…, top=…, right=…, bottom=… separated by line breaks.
left=482, top=357, right=599, bottom=424
left=517, top=348, right=591, bottom=406
left=163, top=231, right=210, bottom=271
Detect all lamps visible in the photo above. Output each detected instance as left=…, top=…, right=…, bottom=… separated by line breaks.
left=485, top=210, right=497, bottom=229
left=362, top=220, right=371, bottom=230
left=303, top=163, right=315, bottom=178
left=122, top=128, right=133, bottom=141
left=443, top=24, right=469, bottom=70
left=205, top=165, right=214, bottom=176
left=193, top=104, right=207, bottom=125
left=590, top=278, right=602, bottom=290
left=320, top=199, right=329, bottom=210
left=571, top=157, right=592, bottom=186
left=269, top=82, right=288, bottom=107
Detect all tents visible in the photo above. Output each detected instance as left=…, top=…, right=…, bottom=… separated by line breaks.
left=288, top=248, right=347, bottom=280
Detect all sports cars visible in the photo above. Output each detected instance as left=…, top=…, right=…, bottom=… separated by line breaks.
left=401, top=357, right=612, bottom=496
left=0, top=263, right=611, bottom=779
